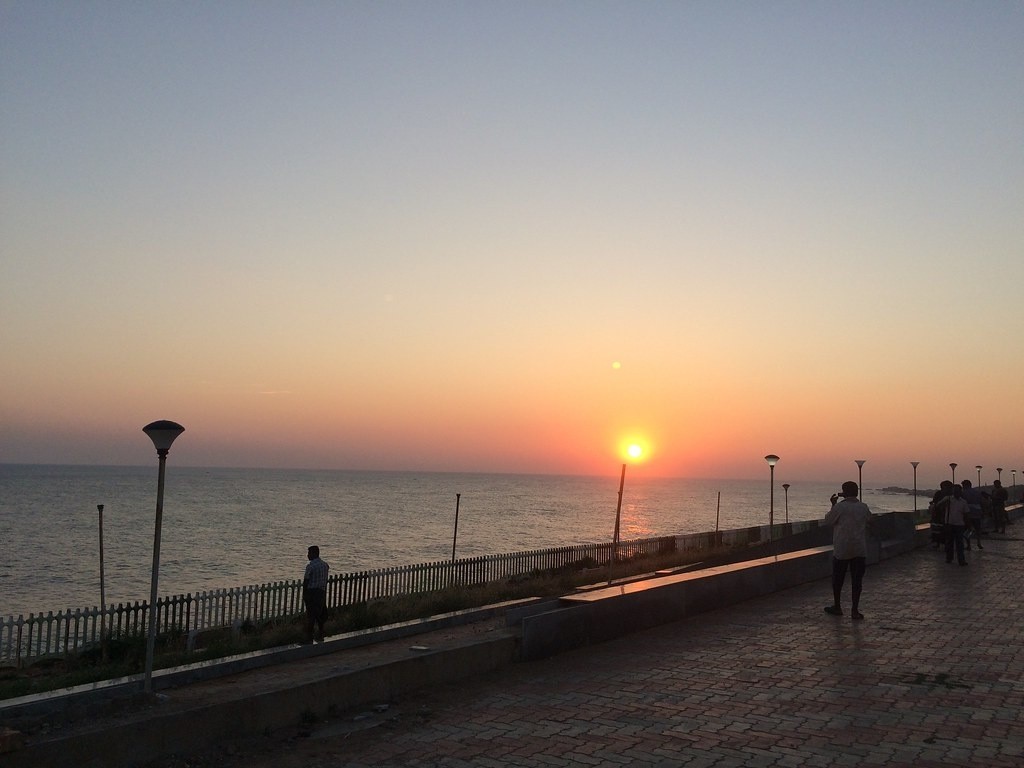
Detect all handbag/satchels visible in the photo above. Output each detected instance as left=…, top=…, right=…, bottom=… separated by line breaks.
left=944, top=523, right=953, bottom=539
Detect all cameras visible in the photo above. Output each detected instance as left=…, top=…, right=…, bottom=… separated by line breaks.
left=838, top=493, right=844, bottom=497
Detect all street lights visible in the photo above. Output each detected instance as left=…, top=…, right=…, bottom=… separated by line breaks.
left=1011, top=470, right=1016, bottom=486
left=855, top=458, right=866, bottom=503
left=949, top=462, right=958, bottom=484
left=765, top=455, right=780, bottom=546
left=782, top=484, right=791, bottom=525
left=911, top=461, right=921, bottom=512
left=142, top=420, right=185, bottom=695
left=976, top=465, right=983, bottom=488
left=997, top=467, right=1003, bottom=482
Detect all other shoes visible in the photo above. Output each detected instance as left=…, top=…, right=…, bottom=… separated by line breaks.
left=958, top=558, right=964, bottom=566
left=945, top=557, right=953, bottom=563
left=851, top=614, right=862, bottom=619
left=824, top=607, right=843, bottom=616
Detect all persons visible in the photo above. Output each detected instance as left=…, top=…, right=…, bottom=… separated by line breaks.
left=298, top=546, right=329, bottom=644
left=824, top=481, right=871, bottom=620
left=929, top=479, right=1008, bottom=565
